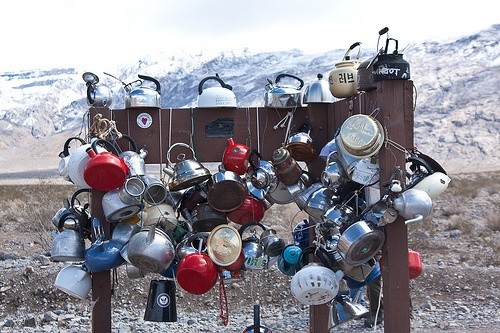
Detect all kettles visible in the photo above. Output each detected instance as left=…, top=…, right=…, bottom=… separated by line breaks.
left=366, top=27, right=411, bottom=84
left=357, top=54, right=378, bottom=91
left=50, top=107, right=451, bottom=333
left=197, top=72, right=236, bottom=108
left=302, top=74, right=336, bottom=104
left=83, top=72, right=113, bottom=108
left=263, top=74, right=303, bottom=109
left=328, top=42, right=363, bottom=98
left=123, top=75, right=160, bottom=109
